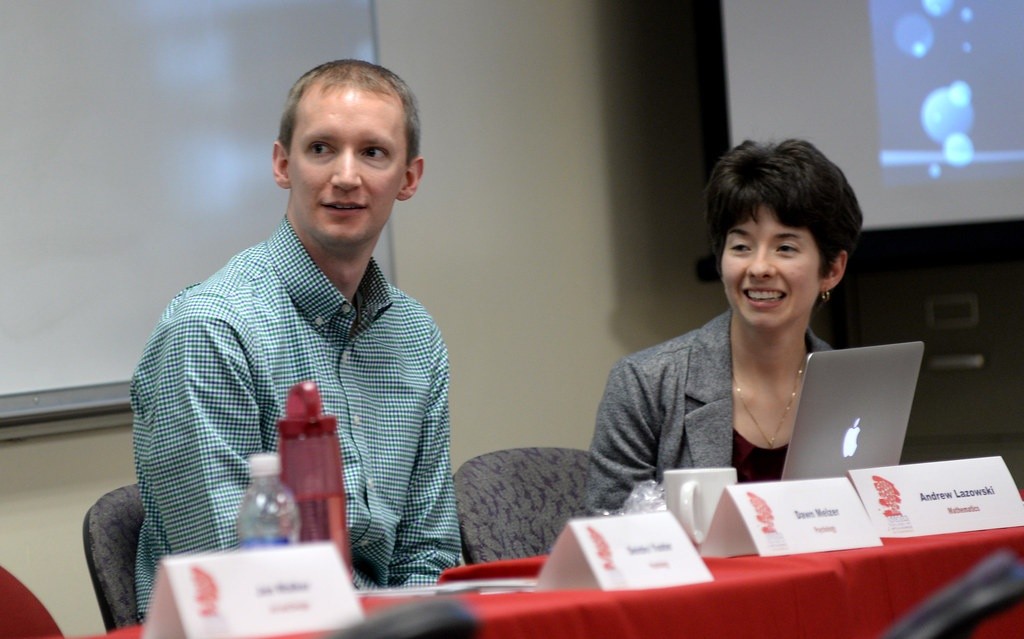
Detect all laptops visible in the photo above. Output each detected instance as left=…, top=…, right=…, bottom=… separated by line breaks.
left=782, top=342, right=925, bottom=482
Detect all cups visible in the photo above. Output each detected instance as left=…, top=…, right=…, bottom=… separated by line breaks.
left=663, top=467, right=737, bottom=553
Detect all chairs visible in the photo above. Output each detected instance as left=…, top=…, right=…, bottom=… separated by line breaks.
left=453, top=446, right=589, bottom=565
left=82, top=483, right=144, bottom=633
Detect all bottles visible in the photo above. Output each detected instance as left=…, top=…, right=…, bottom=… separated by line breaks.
left=237, top=455, right=299, bottom=553
left=279, top=385, right=351, bottom=577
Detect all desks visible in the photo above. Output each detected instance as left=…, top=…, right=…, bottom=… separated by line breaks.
left=82, top=489, right=1024, bottom=638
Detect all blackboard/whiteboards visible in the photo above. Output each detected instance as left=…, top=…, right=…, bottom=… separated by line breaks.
left=0, top=0, right=398, bottom=446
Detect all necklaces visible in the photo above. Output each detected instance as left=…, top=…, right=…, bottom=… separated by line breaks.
left=733, top=353, right=810, bottom=449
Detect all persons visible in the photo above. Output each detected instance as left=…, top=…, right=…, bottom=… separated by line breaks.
left=587, top=136, right=863, bottom=516
left=128, top=59, right=462, bottom=630
